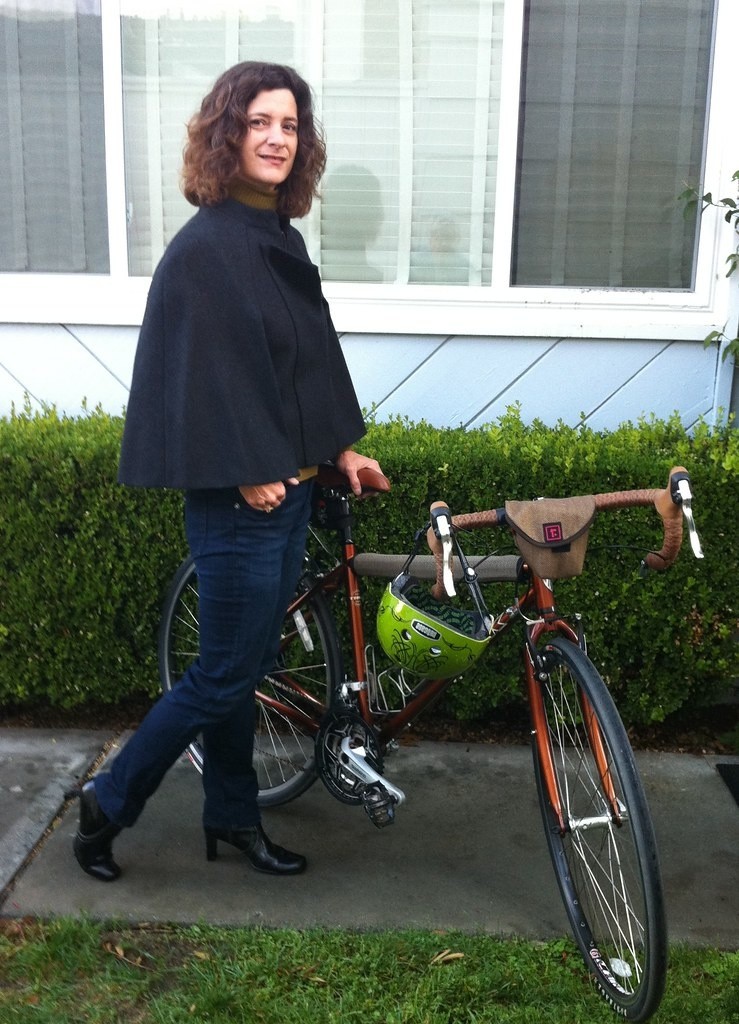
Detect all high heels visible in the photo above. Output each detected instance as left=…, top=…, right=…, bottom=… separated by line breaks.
left=64, top=779, right=121, bottom=881
left=203, top=821, right=307, bottom=875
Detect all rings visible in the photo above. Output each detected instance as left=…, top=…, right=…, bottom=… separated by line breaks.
left=263, top=506, right=273, bottom=513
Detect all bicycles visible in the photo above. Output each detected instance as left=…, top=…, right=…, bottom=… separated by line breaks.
left=157, top=455, right=705, bottom=1024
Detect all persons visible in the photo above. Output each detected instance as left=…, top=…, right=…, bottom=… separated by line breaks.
left=62, top=61, right=386, bottom=881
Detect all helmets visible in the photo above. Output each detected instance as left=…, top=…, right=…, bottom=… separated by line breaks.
left=376, top=572, right=495, bottom=679
